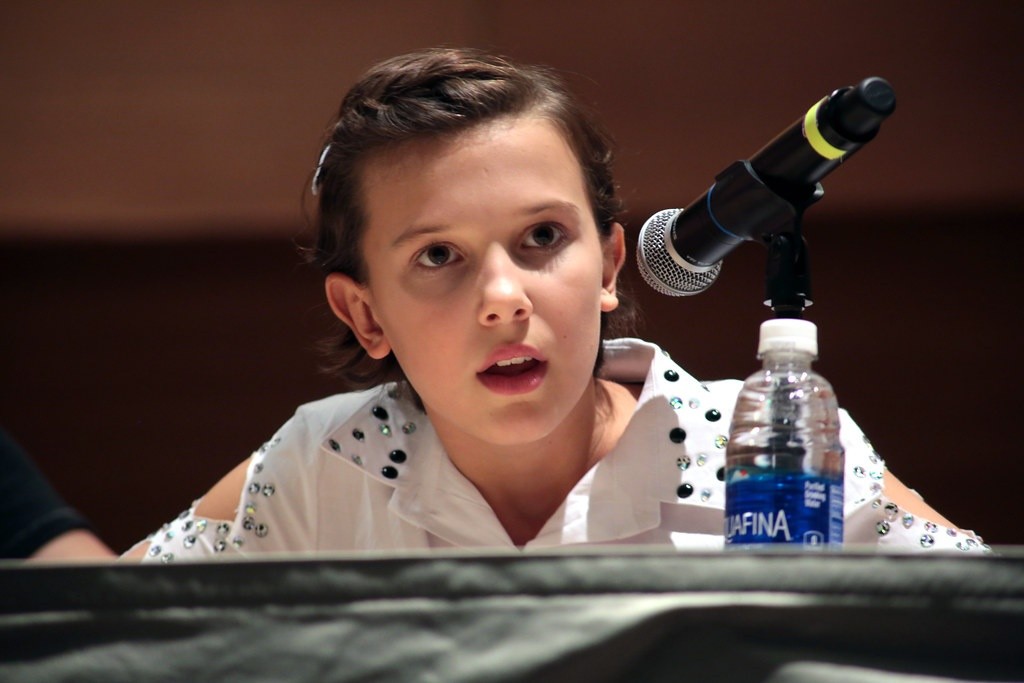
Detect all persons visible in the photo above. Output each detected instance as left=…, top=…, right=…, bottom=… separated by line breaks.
left=109, top=48, right=991, bottom=563
left=0, top=424, right=118, bottom=563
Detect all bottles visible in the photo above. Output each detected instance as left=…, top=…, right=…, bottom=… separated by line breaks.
left=725, top=318, right=845, bottom=551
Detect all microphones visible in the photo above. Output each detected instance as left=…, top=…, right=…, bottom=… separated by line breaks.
left=635, top=78, right=897, bottom=297
